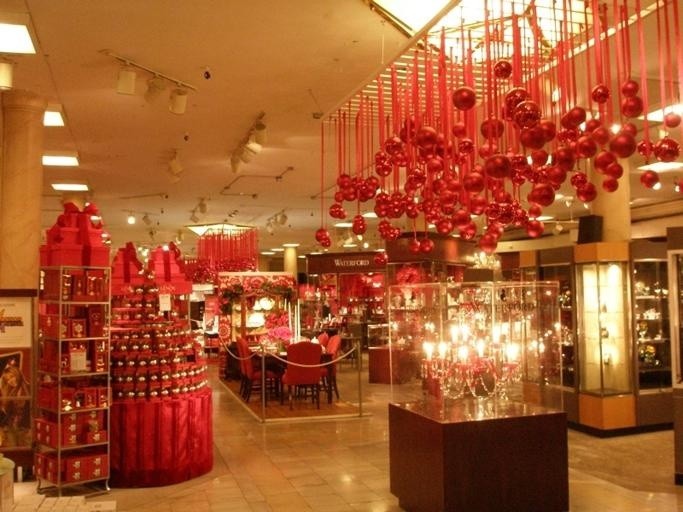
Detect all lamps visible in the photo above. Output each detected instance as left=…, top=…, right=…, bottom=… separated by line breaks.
left=100, top=48, right=197, bottom=115
left=190, top=197, right=208, bottom=223
left=125, top=211, right=153, bottom=225
left=229, top=110, right=268, bottom=179
left=266, top=209, right=288, bottom=235
left=167, top=149, right=182, bottom=177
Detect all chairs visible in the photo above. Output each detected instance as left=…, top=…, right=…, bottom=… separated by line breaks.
left=236, top=334, right=342, bottom=410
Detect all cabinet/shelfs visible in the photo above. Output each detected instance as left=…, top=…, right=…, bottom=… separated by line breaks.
left=31, top=265, right=112, bottom=499
left=109, top=288, right=212, bottom=488
left=494, top=240, right=672, bottom=438
